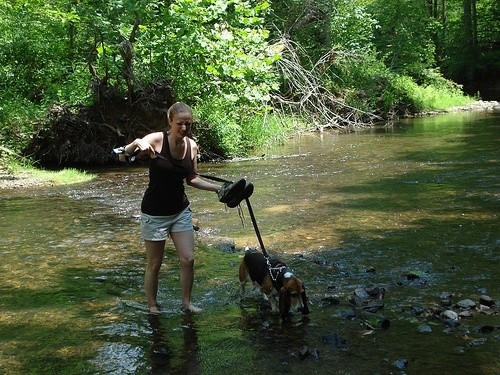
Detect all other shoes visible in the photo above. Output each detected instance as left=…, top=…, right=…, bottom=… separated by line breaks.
left=226, top=183, right=254, bottom=207
left=216, top=178, right=246, bottom=203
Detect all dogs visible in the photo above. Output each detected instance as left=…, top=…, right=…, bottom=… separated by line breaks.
left=238, top=247, right=311, bottom=318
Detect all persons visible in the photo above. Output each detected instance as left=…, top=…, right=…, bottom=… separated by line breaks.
left=119, top=102, right=222, bottom=312
